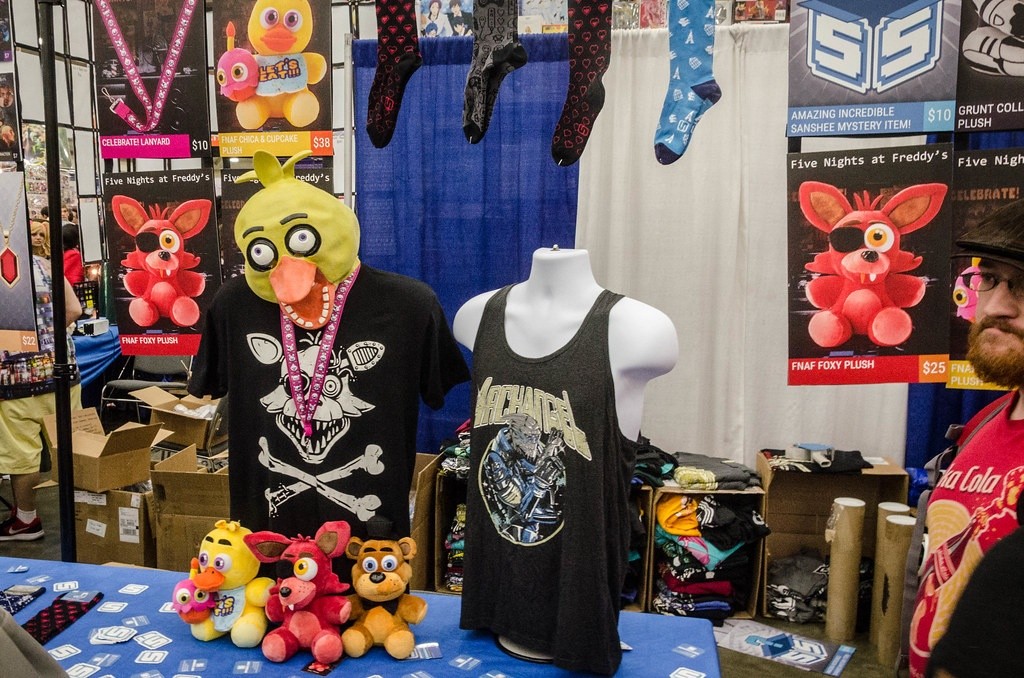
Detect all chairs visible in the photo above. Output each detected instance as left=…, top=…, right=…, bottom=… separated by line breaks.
left=99, top=334, right=193, bottom=424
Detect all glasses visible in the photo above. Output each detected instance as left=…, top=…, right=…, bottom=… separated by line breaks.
left=958, top=271, right=1024, bottom=298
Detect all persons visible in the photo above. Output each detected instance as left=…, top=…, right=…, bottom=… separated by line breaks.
left=30, top=205, right=85, bottom=287
left=921, top=489, right=1024, bottom=678
left=186, top=148, right=471, bottom=596
left=0, top=253, right=83, bottom=541
left=451, top=246, right=678, bottom=678
left=906, top=198, right=1024, bottom=678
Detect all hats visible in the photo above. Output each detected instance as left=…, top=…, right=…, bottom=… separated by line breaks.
left=950, top=197, right=1024, bottom=270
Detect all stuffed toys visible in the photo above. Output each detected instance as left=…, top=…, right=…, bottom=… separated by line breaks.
left=341, top=536, right=427, bottom=659
left=244, top=521, right=352, bottom=665
left=171, top=519, right=276, bottom=649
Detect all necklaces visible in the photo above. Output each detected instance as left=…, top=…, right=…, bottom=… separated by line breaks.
left=0, top=177, right=24, bottom=287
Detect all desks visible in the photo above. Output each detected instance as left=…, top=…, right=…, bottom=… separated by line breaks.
left=0, top=556, right=721, bottom=678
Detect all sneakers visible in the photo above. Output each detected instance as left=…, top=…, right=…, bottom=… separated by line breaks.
left=0, top=505, right=44, bottom=540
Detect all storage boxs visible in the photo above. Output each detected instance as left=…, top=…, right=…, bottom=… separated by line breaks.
left=32, top=387, right=908, bottom=621
